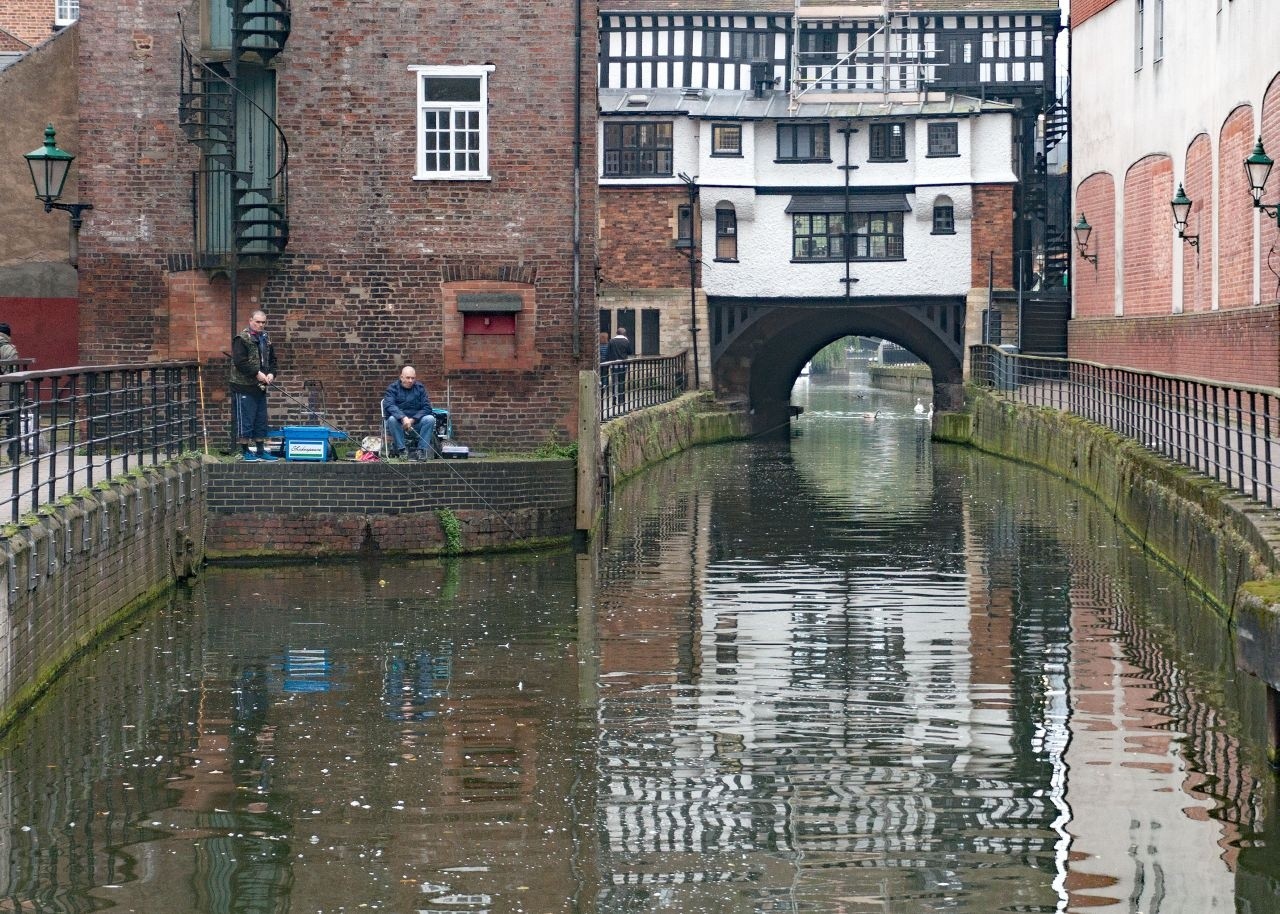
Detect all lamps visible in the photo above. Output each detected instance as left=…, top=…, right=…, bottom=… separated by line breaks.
left=1244, top=133, right=1280, bottom=228
left=1172, top=182, right=1199, bottom=253
left=24, top=123, right=93, bottom=217
left=1073, top=212, right=1097, bottom=265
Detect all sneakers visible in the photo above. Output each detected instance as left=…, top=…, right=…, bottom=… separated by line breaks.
left=242, top=453, right=260, bottom=463
left=255, top=450, right=278, bottom=462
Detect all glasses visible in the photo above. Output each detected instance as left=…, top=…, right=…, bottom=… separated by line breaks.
left=254, top=320, right=265, bottom=324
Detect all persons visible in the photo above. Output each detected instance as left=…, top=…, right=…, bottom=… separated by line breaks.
left=0, top=323, right=18, bottom=468
left=384, top=366, right=435, bottom=462
left=600, top=327, right=632, bottom=407
left=228, top=310, right=279, bottom=461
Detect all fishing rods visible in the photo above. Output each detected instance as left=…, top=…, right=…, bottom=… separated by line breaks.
left=220, top=349, right=452, bottom=512
left=410, top=425, right=542, bottom=560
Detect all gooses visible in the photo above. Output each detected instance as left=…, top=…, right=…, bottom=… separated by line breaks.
left=914, top=398, right=924, bottom=409
left=928, top=402, right=934, bottom=419
left=863, top=410, right=881, bottom=418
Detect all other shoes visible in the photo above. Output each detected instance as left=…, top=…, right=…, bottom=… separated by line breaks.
left=415, top=450, right=427, bottom=462
left=399, top=451, right=409, bottom=461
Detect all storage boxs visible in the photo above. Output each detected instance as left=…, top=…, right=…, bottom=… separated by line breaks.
left=266, top=424, right=347, bottom=462
left=442, top=446, right=469, bottom=460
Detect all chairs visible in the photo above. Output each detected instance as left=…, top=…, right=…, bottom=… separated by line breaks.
left=380, top=399, right=429, bottom=462
